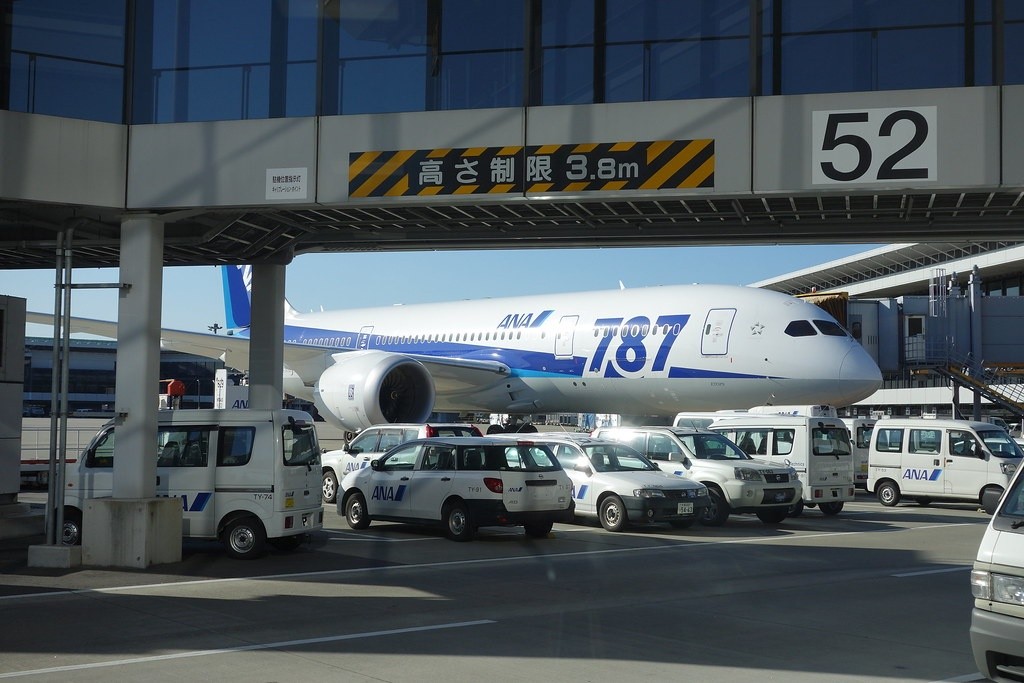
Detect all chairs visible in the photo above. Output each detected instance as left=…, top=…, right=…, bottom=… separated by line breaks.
left=157, top=440, right=203, bottom=468
left=433, top=450, right=481, bottom=470
left=961, top=440, right=973, bottom=456
left=741, top=436, right=770, bottom=456
left=591, top=453, right=604, bottom=466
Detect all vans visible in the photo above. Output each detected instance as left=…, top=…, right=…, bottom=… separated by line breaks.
left=969, top=458, right=1024, bottom=680
left=867, top=419, right=1024, bottom=506
left=44, top=409, right=324, bottom=559
left=673, top=405, right=888, bottom=515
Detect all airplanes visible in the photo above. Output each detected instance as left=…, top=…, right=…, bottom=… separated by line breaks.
left=26, top=266, right=884, bottom=433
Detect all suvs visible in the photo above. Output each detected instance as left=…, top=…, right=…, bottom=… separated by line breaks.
left=588, top=426, right=803, bottom=526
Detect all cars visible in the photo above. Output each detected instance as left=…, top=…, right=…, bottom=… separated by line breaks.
left=505, top=438, right=710, bottom=531
left=321, top=424, right=483, bottom=503
left=336, top=437, right=573, bottom=540
